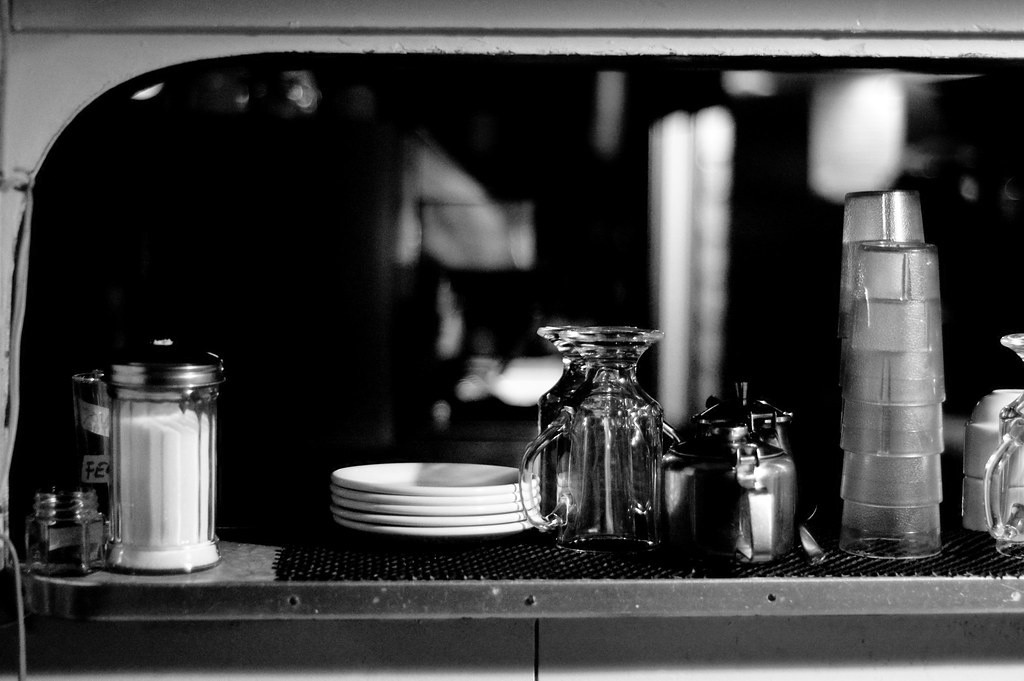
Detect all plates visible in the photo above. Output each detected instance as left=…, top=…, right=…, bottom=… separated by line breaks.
left=329, top=463, right=541, bottom=536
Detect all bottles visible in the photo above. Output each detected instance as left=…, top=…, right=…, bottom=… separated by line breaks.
left=24, top=487, right=108, bottom=578
left=106, top=344, right=225, bottom=576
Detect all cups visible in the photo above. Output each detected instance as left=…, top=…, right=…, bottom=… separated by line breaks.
left=518, top=324, right=682, bottom=558
left=983, top=334, right=1024, bottom=558
left=961, top=389, right=1024, bottom=532
left=834, top=188, right=946, bottom=560
